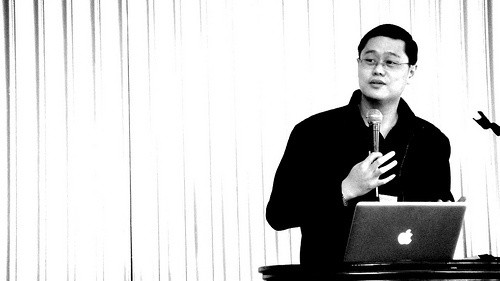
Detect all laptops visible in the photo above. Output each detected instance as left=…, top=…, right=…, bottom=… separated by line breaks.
left=344, top=202, right=466, bottom=266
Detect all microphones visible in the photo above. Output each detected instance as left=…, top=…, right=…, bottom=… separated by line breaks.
left=366, top=109, right=384, bottom=164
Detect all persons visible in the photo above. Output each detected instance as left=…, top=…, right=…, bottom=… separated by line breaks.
left=265, top=23, right=455, bottom=264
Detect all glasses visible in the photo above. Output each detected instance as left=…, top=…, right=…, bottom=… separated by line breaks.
left=356, top=57, right=410, bottom=67
